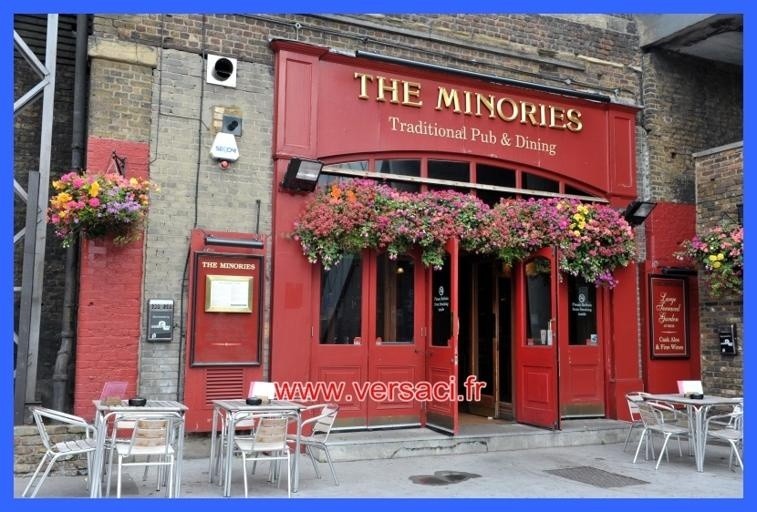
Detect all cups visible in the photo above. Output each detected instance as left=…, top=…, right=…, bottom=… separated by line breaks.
left=684, top=393, right=691, bottom=401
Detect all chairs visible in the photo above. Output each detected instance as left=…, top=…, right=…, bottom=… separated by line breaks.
left=21, top=400, right=339, bottom=499
left=626, top=391, right=743, bottom=472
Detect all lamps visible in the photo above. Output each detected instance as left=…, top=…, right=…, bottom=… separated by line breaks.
left=627, top=200, right=658, bottom=226
left=280, top=157, right=324, bottom=194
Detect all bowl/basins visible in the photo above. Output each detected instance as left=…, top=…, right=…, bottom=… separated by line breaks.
left=128, top=398, right=147, bottom=406
left=691, top=394, right=704, bottom=399
left=245, top=398, right=260, bottom=405
